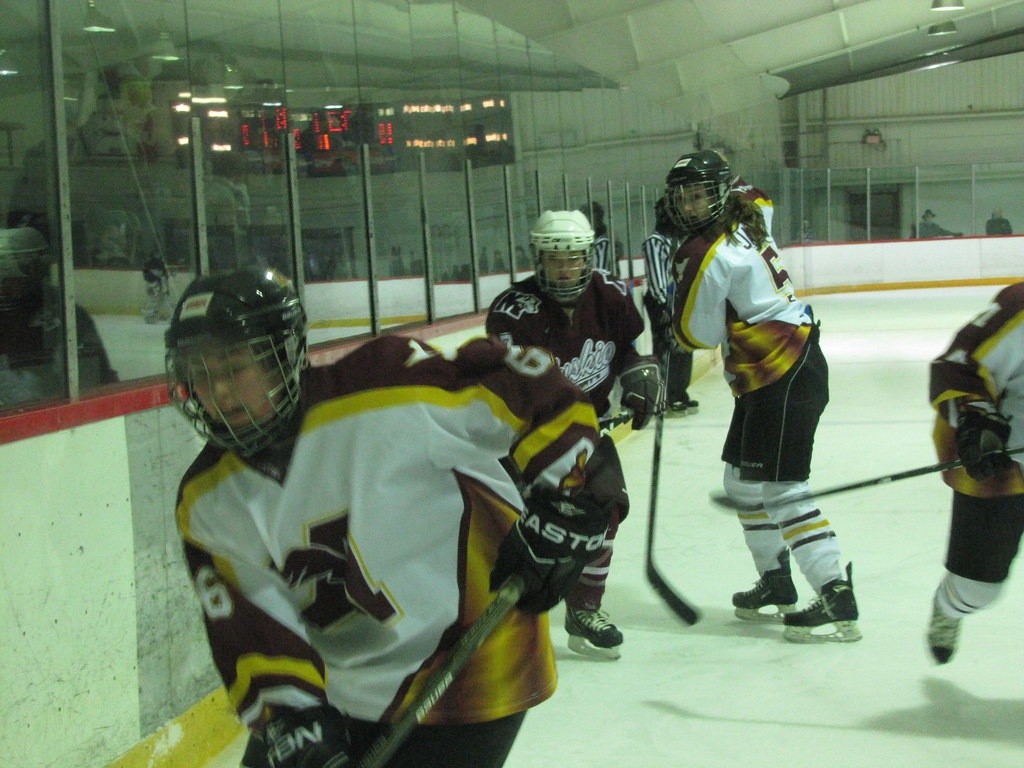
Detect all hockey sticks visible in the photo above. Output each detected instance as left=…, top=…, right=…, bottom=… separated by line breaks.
left=711, top=446, right=1024, bottom=513
left=643, top=339, right=704, bottom=629
left=355, top=570, right=526, bottom=768
left=585, top=409, right=631, bottom=439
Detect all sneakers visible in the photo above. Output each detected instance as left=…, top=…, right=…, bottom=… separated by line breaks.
left=780, top=562, right=862, bottom=644
left=928, top=585, right=962, bottom=666
left=733, top=546, right=798, bottom=625
left=666, top=395, right=699, bottom=418
left=565, top=600, right=624, bottom=663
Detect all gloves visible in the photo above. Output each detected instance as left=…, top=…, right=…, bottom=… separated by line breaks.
left=957, top=402, right=1013, bottom=481
left=653, top=303, right=672, bottom=329
left=488, top=482, right=616, bottom=617
left=618, top=353, right=663, bottom=429
left=244, top=688, right=351, bottom=768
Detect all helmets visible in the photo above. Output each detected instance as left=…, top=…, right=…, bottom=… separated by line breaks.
left=165, top=264, right=305, bottom=458
left=0, top=227, right=52, bottom=323
left=530, top=209, right=593, bottom=303
left=665, top=150, right=730, bottom=232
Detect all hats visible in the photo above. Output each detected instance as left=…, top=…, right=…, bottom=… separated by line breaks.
left=922, top=209, right=936, bottom=219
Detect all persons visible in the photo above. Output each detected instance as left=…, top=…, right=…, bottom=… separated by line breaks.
left=0, top=227, right=115, bottom=409
left=641, top=196, right=698, bottom=410
left=912, top=209, right=963, bottom=236
left=163, top=262, right=600, bottom=768
left=797, top=218, right=814, bottom=243
left=580, top=201, right=619, bottom=277
left=986, top=208, right=1012, bottom=235
left=442, top=264, right=468, bottom=281
left=142, top=254, right=170, bottom=323
left=487, top=210, right=658, bottom=662
left=927, top=280, right=1024, bottom=664
left=478, top=246, right=529, bottom=273
left=665, top=150, right=861, bottom=644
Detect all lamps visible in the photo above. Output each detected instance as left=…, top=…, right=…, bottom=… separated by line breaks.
left=0, top=45, right=20, bottom=75
left=932, top=0, right=965, bottom=11
left=151, top=18, right=179, bottom=60
left=928, top=21, right=957, bottom=36
left=81, top=1, right=116, bottom=33
